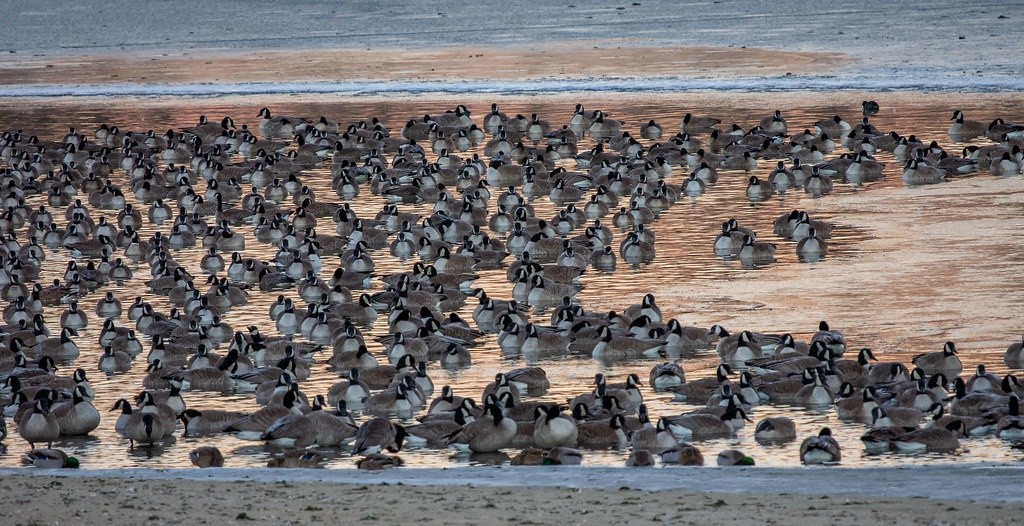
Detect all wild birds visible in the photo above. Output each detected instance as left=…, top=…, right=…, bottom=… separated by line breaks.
left=682, top=100, right=1024, bottom=468
left=0, top=104, right=705, bottom=469
